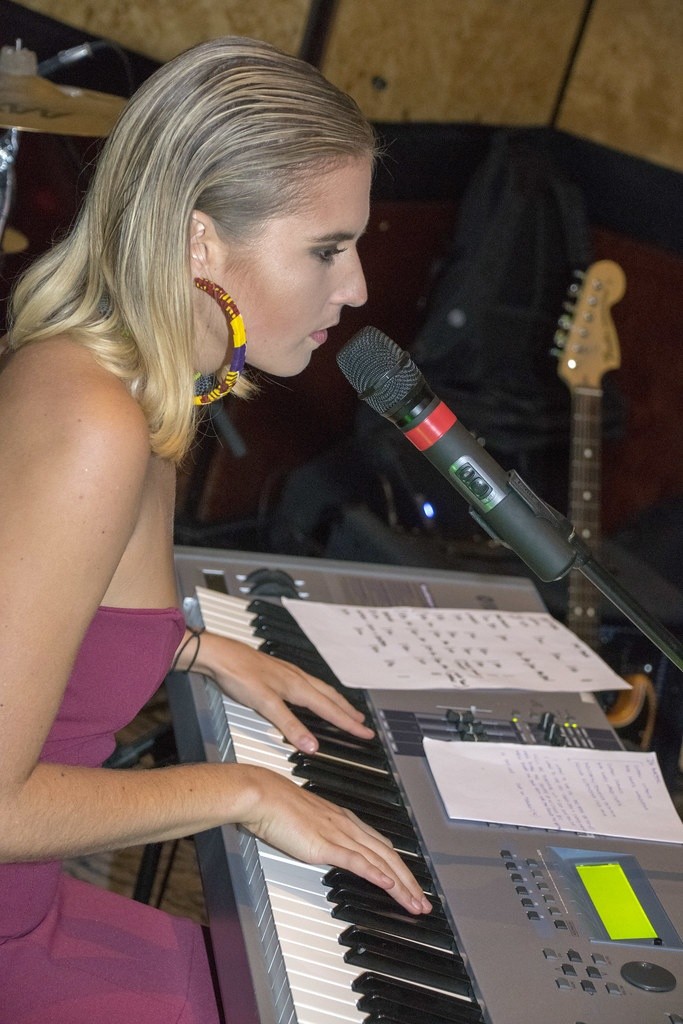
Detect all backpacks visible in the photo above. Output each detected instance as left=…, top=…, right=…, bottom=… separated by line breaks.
left=350, top=133, right=624, bottom=543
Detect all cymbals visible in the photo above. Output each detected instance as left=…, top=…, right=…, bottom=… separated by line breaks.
left=0, top=75, right=130, bottom=137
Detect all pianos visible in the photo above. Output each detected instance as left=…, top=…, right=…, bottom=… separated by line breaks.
left=167, top=541, right=682, bottom=1022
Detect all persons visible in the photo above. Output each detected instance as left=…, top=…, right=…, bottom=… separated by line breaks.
left=0, top=35, right=433, bottom=1024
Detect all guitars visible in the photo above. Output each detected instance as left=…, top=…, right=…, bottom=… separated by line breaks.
left=551, top=254, right=673, bottom=757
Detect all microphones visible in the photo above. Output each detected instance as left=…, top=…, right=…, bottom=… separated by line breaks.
left=36, top=42, right=95, bottom=74
left=335, top=326, right=577, bottom=582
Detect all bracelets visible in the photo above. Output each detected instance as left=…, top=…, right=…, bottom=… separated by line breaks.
left=172, top=626, right=205, bottom=673
